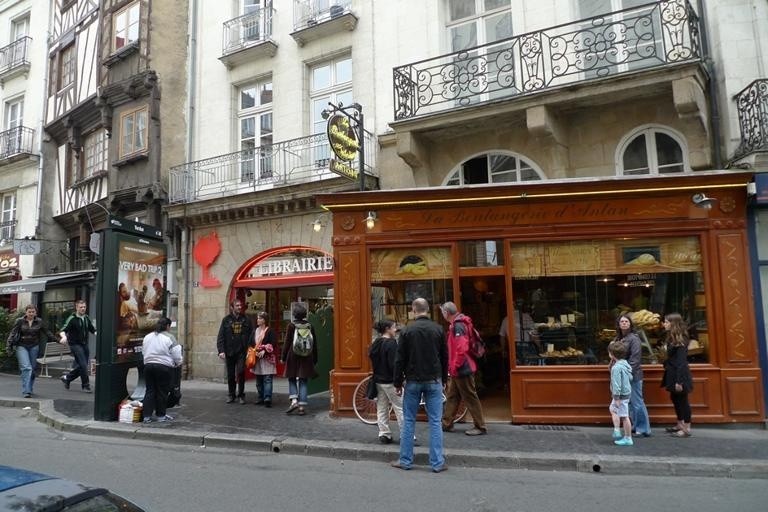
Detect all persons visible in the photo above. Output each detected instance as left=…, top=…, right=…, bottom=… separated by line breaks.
left=54, top=300, right=97, bottom=394
left=367, top=319, right=408, bottom=444
left=609, top=314, right=654, bottom=436
left=603, top=341, right=636, bottom=447
left=500, top=301, right=546, bottom=370
left=391, top=297, right=451, bottom=475
left=140, top=312, right=183, bottom=424
left=6, top=305, right=67, bottom=398
left=658, top=312, right=698, bottom=440
left=114, top=276, right=165, bottom=346
left=435, top=302, right=492, bottom=435
left=216, top=298, right=319, bottom=417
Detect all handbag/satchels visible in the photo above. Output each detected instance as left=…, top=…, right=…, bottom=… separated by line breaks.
left=366, top=377, right=377, bottom=400
left=12, top=329, right=20, bottom=344
left=244, top=347, right=255, bottom=369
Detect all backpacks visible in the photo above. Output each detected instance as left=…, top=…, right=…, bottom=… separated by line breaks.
left=468, top=327, right=486, bottom=359
left=290, top=321, right=313, bottom=356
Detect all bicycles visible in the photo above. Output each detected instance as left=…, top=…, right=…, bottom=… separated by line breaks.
left=353, top=371, right=469, bottom=426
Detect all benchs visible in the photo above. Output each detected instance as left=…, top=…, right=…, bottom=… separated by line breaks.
left=35, top=341, right=75, bottom=379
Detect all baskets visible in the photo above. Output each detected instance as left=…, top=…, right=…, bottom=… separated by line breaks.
left=120, top=406, right=142, bottom=422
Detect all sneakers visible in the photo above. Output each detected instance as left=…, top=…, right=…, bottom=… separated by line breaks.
left=379, top=428, right=482, bottom=472
left=226, top=394, right=306, bottom=415
left=23, top=394, right=30, bottom=398
left=157, top=415, right=173, bottom=423
left=60, top=375, right=69, bottom=389
left=612, top=426, right=691, bottom=446
left=82, top=387, right=91, bottom=393
left=143, top=417, right=152, bottom=424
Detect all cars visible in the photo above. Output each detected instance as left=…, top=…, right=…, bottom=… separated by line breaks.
left=0, top=464, right=144, bottom=511
